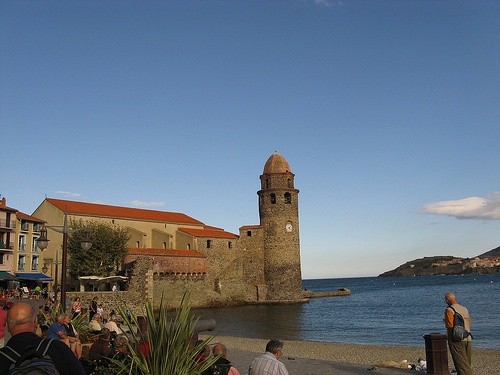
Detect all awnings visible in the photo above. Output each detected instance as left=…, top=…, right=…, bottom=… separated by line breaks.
left=0, top=271, right=18, bottom=281
left=16, top=273, right=54, bottom=281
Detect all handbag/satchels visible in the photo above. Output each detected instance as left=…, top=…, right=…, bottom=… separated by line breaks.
left=450, top=306, right=464, bottom=340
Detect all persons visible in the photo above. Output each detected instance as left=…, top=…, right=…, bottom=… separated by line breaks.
left=0, top=284, right=150, bottom=375
left=444, top=293, right=473, bottom=375
left=248, top=339, right=289, bottom=375
left=190, top=341, right=240, bottom=375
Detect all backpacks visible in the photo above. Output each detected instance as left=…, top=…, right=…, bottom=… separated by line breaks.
left=0, top=339, right=59, bottom=375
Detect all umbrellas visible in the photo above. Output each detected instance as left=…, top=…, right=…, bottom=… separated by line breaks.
left=103, top=275, right=129, bottom=281
left=80, top=276, right=102, bottom=280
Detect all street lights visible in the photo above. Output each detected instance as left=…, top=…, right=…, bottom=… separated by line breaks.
left=40, top=260, right=71, bottom=307
left=34, top=205, right=93, bottom=314
